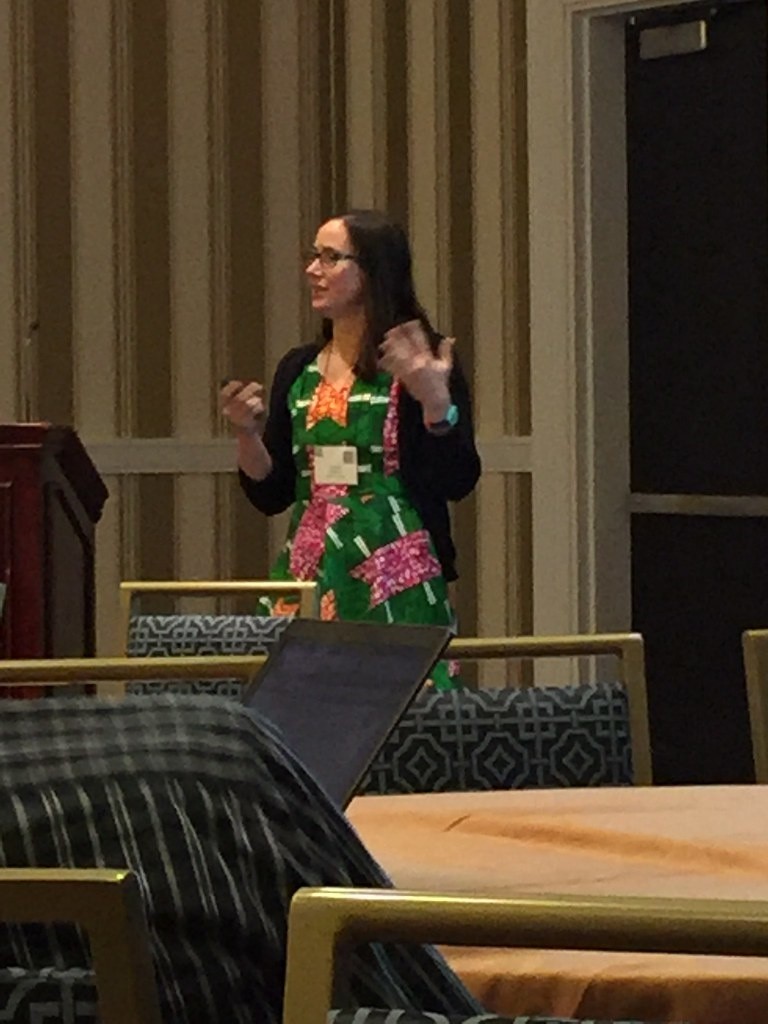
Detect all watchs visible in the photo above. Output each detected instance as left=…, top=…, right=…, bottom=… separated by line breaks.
left=424, top=403, right=459, bottom=431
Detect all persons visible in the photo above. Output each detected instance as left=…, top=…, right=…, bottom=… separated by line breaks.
left=0, top=694, right=488, bottom=1024
left=220, top=213, right=481, bottom=692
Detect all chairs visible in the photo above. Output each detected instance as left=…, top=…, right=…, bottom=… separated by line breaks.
left=351, top=632, right=653, bottom=795
left=0, top=866, right=163, bottom=1024
left=282, top=886, right=768, bottom=1024
left=0, top=578, right=321, bottom=701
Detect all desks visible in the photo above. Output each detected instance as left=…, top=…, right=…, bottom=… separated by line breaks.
left=340, top=782, right=768, bottom=1024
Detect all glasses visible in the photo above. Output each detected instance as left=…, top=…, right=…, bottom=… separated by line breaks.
left=301, top=249, right=358, bottom=269
